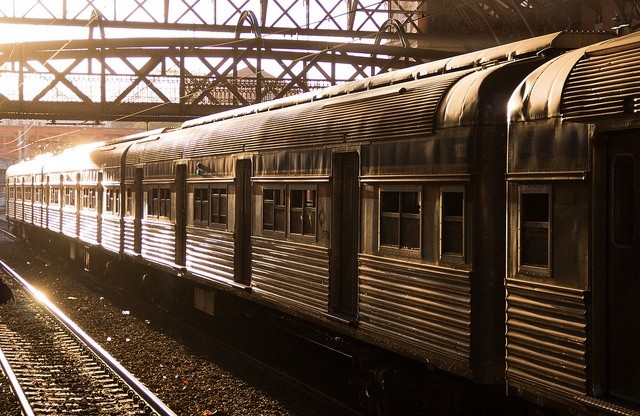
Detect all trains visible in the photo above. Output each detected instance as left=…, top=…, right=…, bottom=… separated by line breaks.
left=5, top=27, right=640, bottom=416
